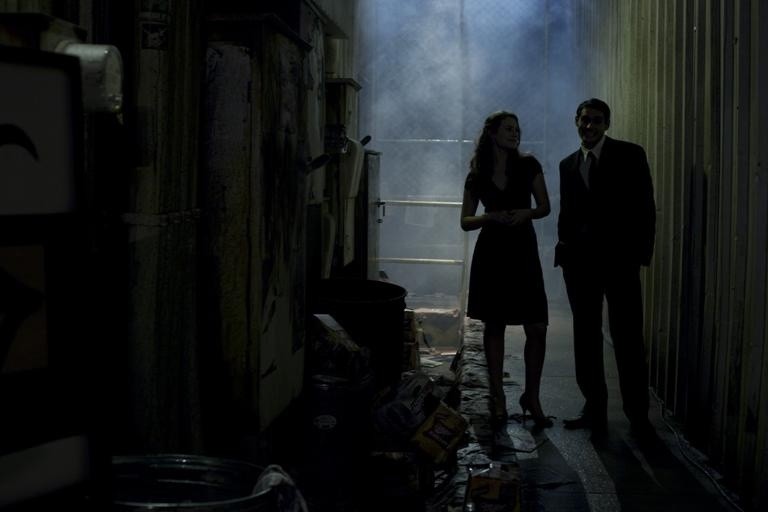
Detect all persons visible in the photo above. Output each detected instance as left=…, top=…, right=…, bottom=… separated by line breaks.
left=459, top=110, right=553, bottom=429
left=554, top=98, right=657, bottom=450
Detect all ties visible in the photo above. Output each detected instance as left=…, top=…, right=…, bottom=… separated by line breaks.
left=589, top=151, right=597, bottom=192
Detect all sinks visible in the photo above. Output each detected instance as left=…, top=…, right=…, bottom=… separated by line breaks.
left=84, top=452, right=280, bottom=509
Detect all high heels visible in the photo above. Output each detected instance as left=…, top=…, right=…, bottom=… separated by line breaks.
left=520, top=392, right=553, bottom=427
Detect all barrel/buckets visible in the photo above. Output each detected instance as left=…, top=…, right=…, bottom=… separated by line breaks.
left=305, top=373, right=371, bottom=458
left=290, top=278, right=407, bottom=370
